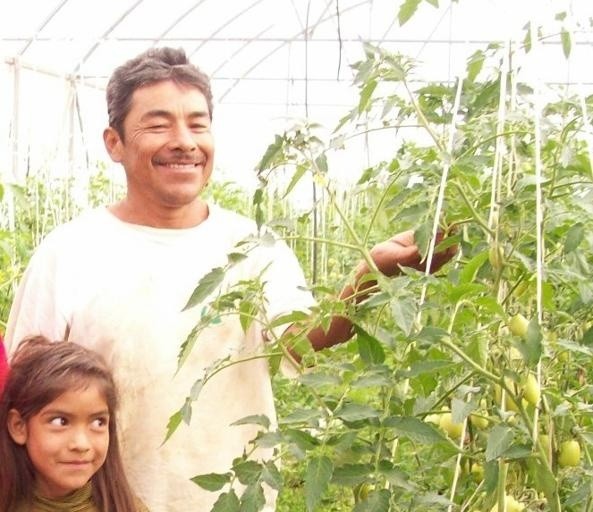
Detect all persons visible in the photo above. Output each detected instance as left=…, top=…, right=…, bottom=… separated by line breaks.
left=1, top=335, right=150, bottom=512
left=1, top=47, right=460, bottom=512
